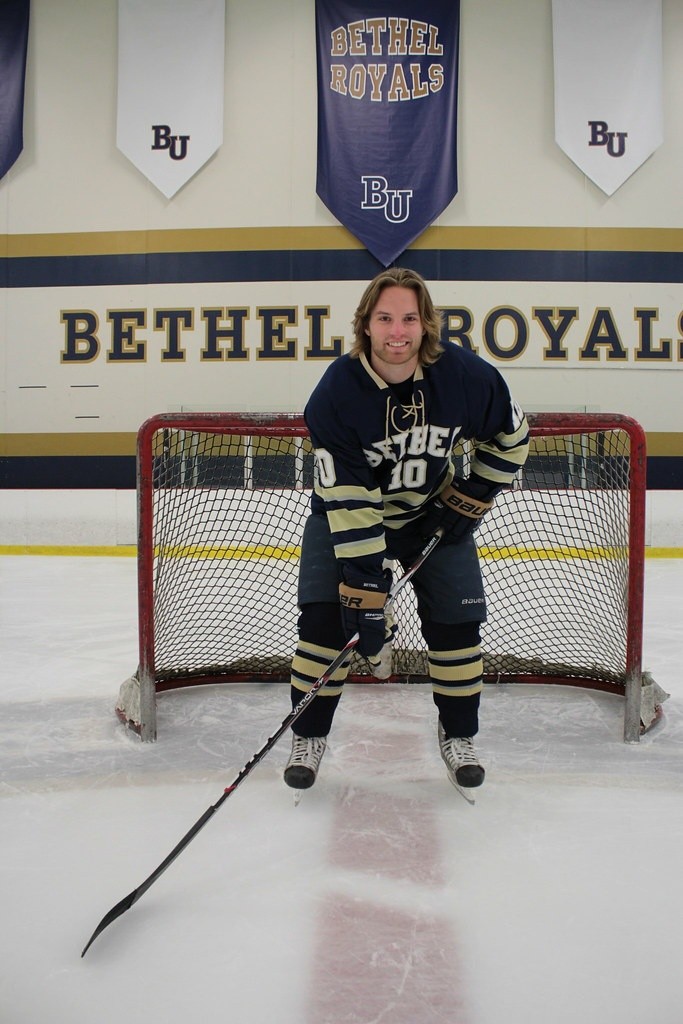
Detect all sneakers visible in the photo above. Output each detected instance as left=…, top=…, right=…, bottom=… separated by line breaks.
left=284, top=734, right=332, bottom=807
left=437, top=714, right=484, bottom=805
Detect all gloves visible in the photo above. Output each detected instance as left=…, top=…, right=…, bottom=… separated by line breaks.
left=417, top=482, right=493, bottom=557
left=338, top=566, right=387, bottom=666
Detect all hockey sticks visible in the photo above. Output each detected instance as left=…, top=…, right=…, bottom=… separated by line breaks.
left=80, top=530, right=448, bottom=962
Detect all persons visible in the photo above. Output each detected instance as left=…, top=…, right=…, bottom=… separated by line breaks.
left=283, top=269, right=530, bottom=789
